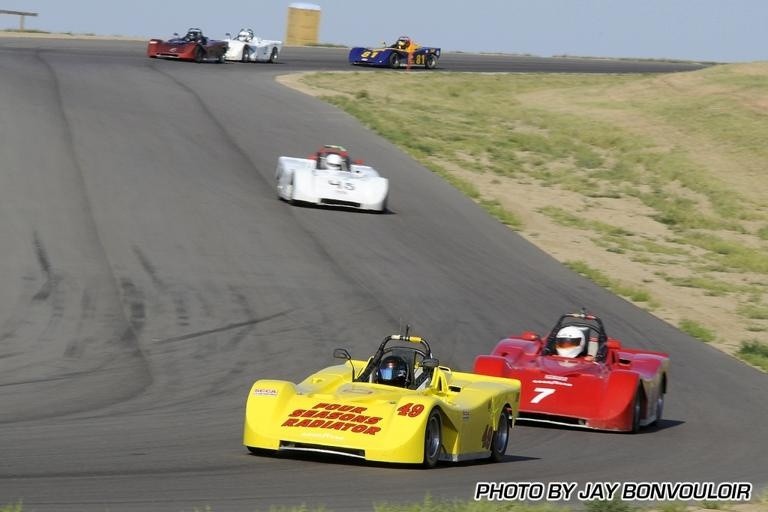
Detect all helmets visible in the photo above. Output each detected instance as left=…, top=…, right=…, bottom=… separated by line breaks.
left=397, top=37, right=406, bottom=47
left=186, top=32, right=195, bottom=41
left=556, top=327, right=585, bottom=358
left=237, top=33, right=248, bottom=42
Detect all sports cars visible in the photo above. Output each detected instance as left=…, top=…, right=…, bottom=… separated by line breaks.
left=348, top=35, right=442, bottom=68
left=243, top=322, right=522, bottom=467
left=472, top=307, right=672, bottom=434
left=147, top=26, right=284, bottom=64
left=276, top=140, right=389, bottom=212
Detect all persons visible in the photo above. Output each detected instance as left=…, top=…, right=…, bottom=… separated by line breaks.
left=396, top=38, right=407, bottom=50
left=184, top=28, right=199, bottom=43
left=238, top=31, right=250, bottom=43
left=553, top=325, right=586, bottom=359
